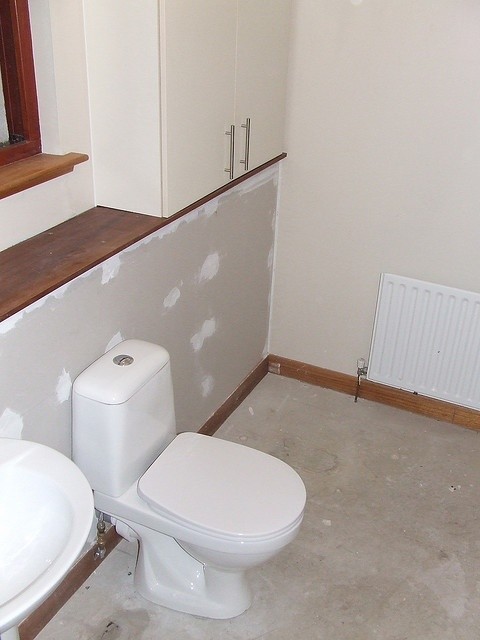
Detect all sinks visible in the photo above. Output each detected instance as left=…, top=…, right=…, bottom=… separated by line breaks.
left=0, top=438, right=94, bottom=636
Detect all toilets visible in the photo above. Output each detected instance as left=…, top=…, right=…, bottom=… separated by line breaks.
left=71, top=339, right=307, bottom=620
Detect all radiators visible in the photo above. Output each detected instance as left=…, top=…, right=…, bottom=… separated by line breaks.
left=366, top=273, right=479, bottom=411
